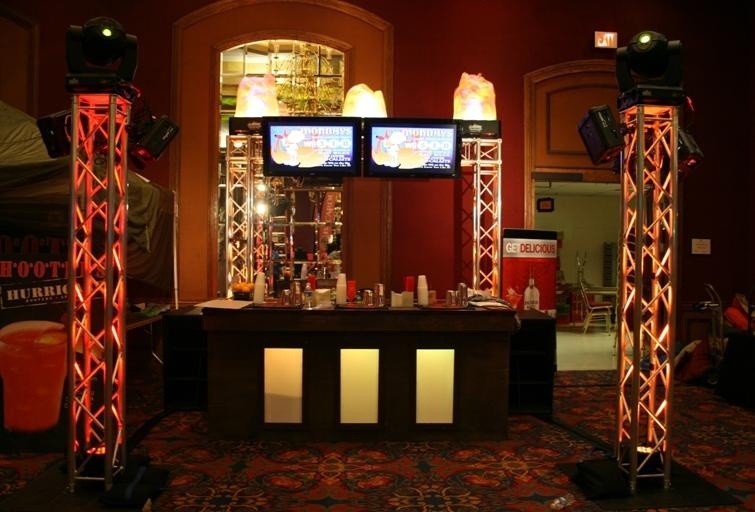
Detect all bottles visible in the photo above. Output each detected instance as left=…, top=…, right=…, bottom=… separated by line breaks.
left=292, top=275, right=318, bottom=309
left=523, top=263, right=541, bottom=311
left=363, top=284, right=385, bottom=307
left=447, top=282, right=469, bottom=308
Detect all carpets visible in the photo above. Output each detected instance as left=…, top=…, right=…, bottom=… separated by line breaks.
left=1, top=368, right=753, bottom=510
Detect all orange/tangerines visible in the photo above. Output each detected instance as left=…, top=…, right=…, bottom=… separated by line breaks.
left=231, top=281, right=255, bottom=293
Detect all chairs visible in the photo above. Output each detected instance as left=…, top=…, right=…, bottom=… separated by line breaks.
left=704, top=283, right=740, bottom=373
left=577, top=278, right=613, bottom=336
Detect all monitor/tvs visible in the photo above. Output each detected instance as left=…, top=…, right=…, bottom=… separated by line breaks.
left=260, top=115, right=361, bottom=178
left=363, top=117, right=463, bottom=179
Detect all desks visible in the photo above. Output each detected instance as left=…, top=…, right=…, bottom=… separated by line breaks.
left=127, top=308, right=165, bottom=367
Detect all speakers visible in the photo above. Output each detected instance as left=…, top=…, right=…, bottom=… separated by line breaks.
left=508, top=316, right=555, bottom=416
left=158, top=313, right=203, bottom=411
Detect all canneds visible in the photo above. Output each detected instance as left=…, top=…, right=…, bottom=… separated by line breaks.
left=447, top=290, right=455, bottom=305
left=457, top=283, right=468, bottom=306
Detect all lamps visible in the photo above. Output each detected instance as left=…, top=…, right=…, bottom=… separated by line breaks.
left=128, top=116, right=179, bottom=171
left=577, top=105, right=627, bottom=165
left=592, top=30, right=619, bottom=48
left=678, top=126, right=701, bottom=179
left=614, top=28, right=691, bottom=105
left=36, top=116, right=72, bottom=159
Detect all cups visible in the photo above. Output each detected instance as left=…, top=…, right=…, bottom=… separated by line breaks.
left=1, top=319, right=67, bottom=431
left=335, top=273, right=356, bottom=304
left=506, top=294, right=521, bottom=310
left=404, top=274, right=436, bottom=307
left=253, top=272, right=266, bottom=304
left=282, top=289, right=291, bottom=305
